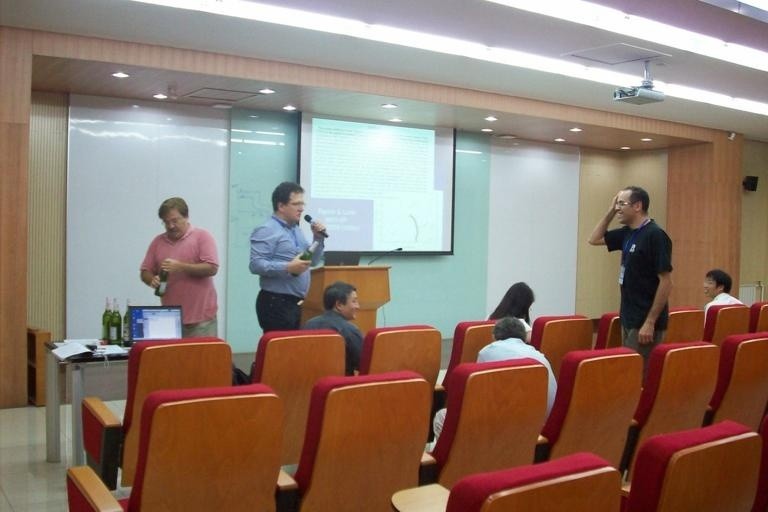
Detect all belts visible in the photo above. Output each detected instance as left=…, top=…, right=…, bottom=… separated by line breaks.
left=266, top=293, right=304, bottom=305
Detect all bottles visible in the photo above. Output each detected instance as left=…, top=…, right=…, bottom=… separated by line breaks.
left=123, top=297, right=132, bottom=345
left=108, top=297, right=122, bottom=344
left=155, top=268, right=168, bottom=296
left=103, top=296, right=113, bottom=339
left=291, top=240, right=319, bottom=277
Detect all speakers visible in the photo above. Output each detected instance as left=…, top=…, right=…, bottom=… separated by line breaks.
left=743, top=176, right=759, bottom=192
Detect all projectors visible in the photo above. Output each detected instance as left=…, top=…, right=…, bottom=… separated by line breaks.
left=614, top=87, right=665, bottom=105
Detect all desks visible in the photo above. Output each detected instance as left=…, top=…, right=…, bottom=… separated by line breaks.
left=43, top=339, right=142, bottom=468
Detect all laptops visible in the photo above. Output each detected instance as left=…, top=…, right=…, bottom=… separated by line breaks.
left=128, top=305, right=184, bottom=342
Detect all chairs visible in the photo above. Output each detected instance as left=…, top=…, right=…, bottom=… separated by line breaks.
left=593, top=304, right=768, bottom=350
left=67, top=381, right=283, bottom=512
left=441, top=319, right=498, bottom=393
left=278, top=369, right=431, bottom=512
left=351, top=324, right=442, bottom=393
left=81, top=338, right=233, bottom=492
left=232, top=331, right=345, bottom=467
left=531, top=315, right=593, bottom=381
left=620, top=419, right=762, bottom=512
left=703, top=332, right=768, bottom=432
left=391, top=452, right=621, bottom=512
left=422, top=358, right=548, bottom=491
left=536, top=347, right=642, bottom=469
left=623, top=340, right=720, bottom=499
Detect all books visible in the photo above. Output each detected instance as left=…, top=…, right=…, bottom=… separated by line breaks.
left=47, top=341, right=128, bottom=363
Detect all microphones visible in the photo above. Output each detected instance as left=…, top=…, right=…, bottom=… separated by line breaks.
left=364, top=247, right=403, bottom=266
left=304, top=214, right=328, bottom=238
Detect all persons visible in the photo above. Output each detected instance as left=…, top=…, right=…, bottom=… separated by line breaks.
left=587, top=186, right=674, bottom=354
left=303, top=282, right=365, bottom=376
left=140, top=198, right=219, bottom=338
left=433, top=315, right=557, bottom=448
left=249, top=182, right=325, bottom=335
left=488, top=282, right=534, bottom=343
left=703, top=269, right=743, bottom=329
